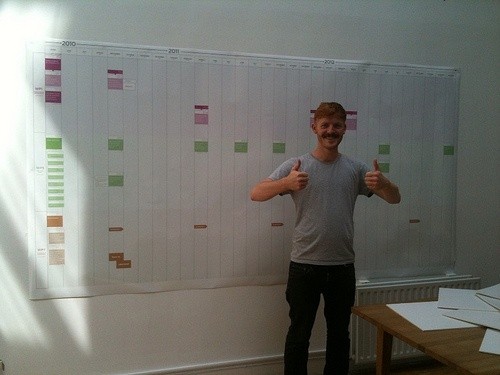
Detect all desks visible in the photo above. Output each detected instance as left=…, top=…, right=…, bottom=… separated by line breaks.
left=351, top=301, right=500, bottom=375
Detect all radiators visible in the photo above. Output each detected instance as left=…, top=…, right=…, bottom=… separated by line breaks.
left=350, top=275, right=480, bottom=363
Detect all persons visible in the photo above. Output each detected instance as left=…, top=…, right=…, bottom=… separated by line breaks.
left=249, top=101, right=402, bottom=375
